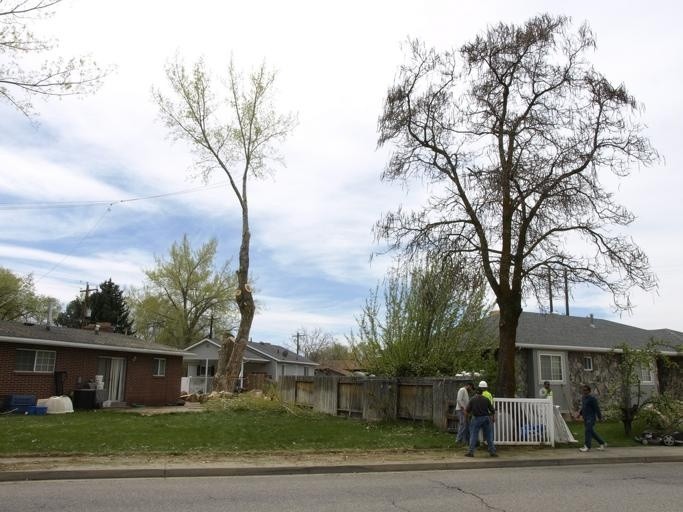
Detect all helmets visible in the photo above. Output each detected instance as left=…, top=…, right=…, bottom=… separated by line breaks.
left=477, top=380, right=488, bottom=387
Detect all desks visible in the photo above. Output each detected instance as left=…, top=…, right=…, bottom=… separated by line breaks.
left=73, top=388, right=97, bottom=414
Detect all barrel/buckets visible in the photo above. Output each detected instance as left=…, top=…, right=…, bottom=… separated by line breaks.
left=88, top=375, right=105, bottom=389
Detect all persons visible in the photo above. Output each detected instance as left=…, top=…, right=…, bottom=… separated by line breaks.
left=538, top=380, right=553, bottom=399
left=575, top=384, right=606, bottom=451
left=454, top=380, right=472, bottom=443
left=463, top=387, right=498, bottom=457
left=476, top=380, right=494, bottom=450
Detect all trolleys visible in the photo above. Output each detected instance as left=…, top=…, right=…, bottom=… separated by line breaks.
left=634, top=415, right=683, bottom=446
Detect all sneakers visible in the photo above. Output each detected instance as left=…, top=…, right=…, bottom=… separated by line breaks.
left=579, top=442, right=607, bottom=452
